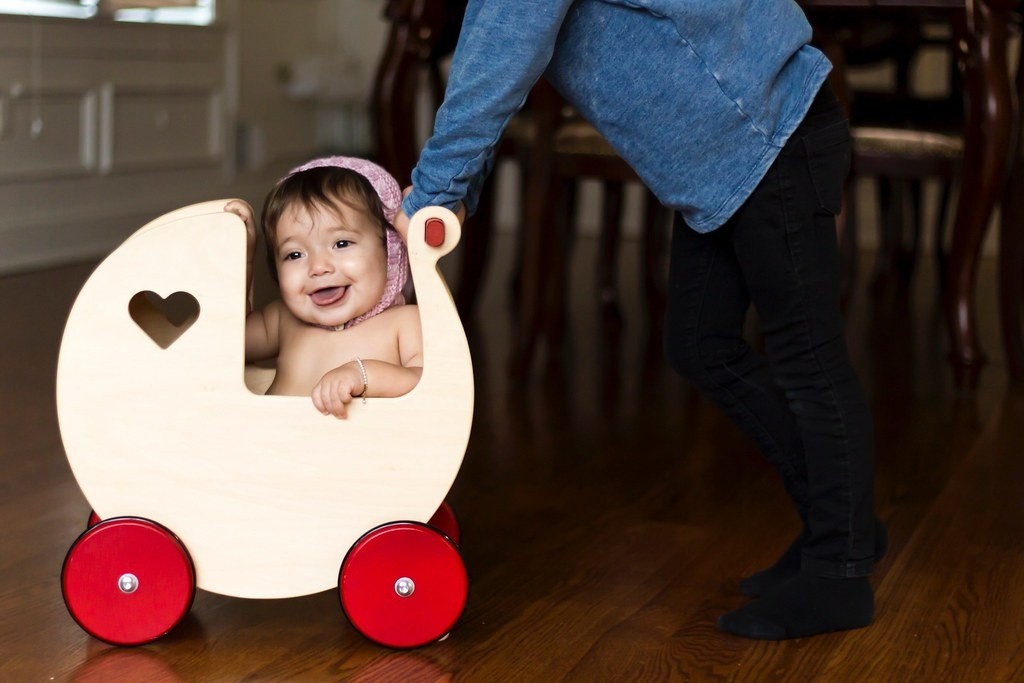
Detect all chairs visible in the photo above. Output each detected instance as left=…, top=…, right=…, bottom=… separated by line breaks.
left=364, top=0, right=1024, bottom=445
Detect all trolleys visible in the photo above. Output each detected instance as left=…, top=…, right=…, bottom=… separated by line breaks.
left=55, top=197, right=475, bottom=649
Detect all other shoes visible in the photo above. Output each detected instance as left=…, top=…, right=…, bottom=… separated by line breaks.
left=715, top=569, right=875, bottom=639
left=739, top=512, right=887, bottom=597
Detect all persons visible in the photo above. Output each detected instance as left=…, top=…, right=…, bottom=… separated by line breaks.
left=223, top=156, right=422, bottom=416
left=393, top=0, right=892, bottom=641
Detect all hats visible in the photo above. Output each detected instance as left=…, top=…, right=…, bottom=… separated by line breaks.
left=275, top=158, right=412, bottom=331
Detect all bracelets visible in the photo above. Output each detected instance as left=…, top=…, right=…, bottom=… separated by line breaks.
left=357, top=359, right=369, bottom=405
